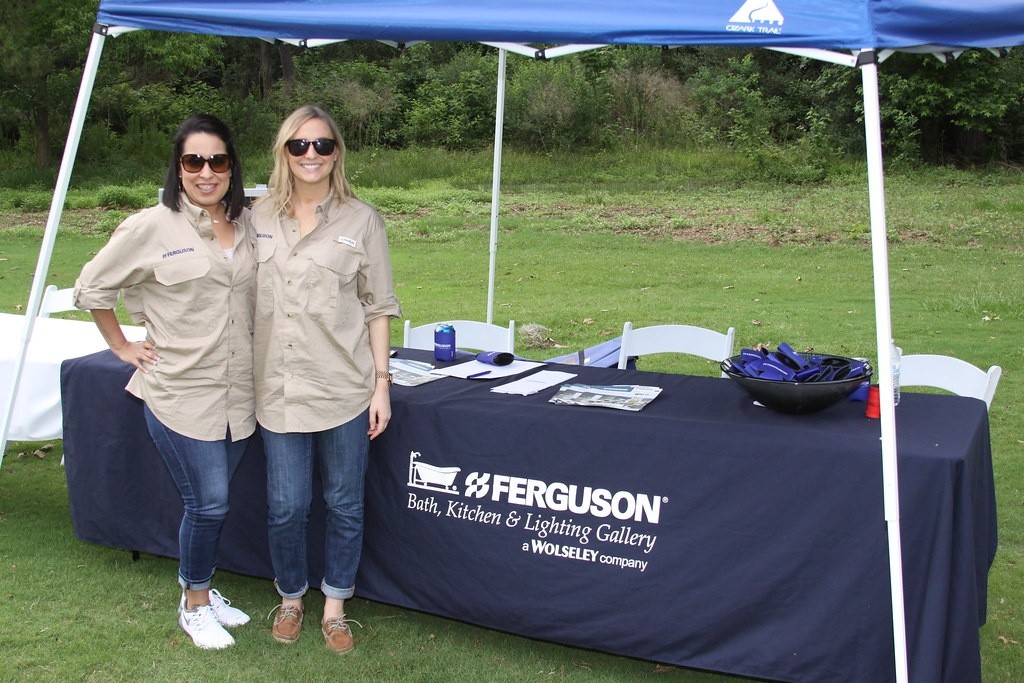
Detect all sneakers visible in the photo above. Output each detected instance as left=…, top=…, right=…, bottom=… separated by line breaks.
left=177, top=588, right=251, bottom=650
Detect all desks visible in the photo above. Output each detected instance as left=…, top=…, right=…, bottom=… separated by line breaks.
left=0, top=313, right=148, bottom=466
left=59, top=345, right=998, bottom=683
left=159, top=188, right=268, bottom=202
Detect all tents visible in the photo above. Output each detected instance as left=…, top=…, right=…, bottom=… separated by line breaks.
left=0, top=1, right=1018, bottom=683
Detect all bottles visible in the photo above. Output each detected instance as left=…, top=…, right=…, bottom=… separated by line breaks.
left=891, top=339, right=901, bottom=407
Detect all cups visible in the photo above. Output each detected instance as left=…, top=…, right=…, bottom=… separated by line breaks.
left=865, top=384, right=880, bottom=418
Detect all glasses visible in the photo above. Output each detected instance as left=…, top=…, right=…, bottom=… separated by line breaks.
left=285, top=138, right=337, bottom=157
left=178, top=154, right=232, bottom=174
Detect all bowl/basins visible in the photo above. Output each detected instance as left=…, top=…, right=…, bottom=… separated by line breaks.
left=720, top=353, right=873, bottom=414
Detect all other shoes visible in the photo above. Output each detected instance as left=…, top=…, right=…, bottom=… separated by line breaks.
left=320, top=613, right=363, bottom=655
left=266, top=599, right=304, bottom=643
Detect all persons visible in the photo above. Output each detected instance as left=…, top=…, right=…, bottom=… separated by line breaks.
left=250, top=102, right=395, bottom=655
left=72, top=110, right=252, bottom=651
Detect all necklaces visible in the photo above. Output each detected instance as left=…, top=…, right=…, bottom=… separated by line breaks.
left=211, top=205, right=221, bottom=226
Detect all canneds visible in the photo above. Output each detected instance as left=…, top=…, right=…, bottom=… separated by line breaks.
left=848, top=358, right=870, bottom=402
left=434, top=324, right=456, bottom=362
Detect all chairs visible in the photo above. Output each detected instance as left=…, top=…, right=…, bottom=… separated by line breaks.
left=37, top=285, right=120, bottom=319
left=403, top=319, right=515, bottom=356
left=618, top=321, right=735, bottom=378
left=877, top=346, right=1002, bottom=411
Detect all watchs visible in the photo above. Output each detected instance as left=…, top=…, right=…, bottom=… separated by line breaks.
left=375, top=370, right=393, bottom=386
